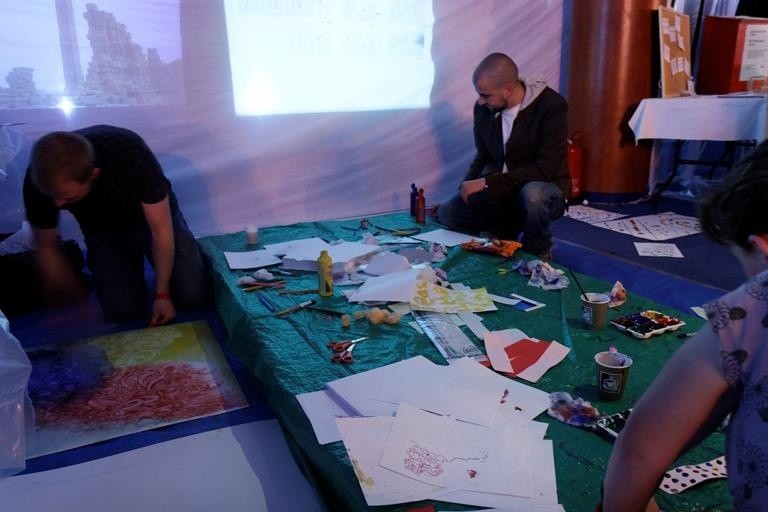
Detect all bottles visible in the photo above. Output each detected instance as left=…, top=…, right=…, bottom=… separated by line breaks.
left=317, top=251, right=334, bottom=297
left=410, top=183, right=419, bottom=216
left=415, top=188, right=425, bottom=223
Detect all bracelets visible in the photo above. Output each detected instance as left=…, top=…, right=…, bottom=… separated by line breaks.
left=155, top=290, right=169, bottom=301
left=484, top=176, right=491, bottom=191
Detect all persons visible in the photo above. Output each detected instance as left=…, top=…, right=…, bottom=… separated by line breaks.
left=603, top=137, right=767, bottom=510
left=437, top=51, right=574, bottom=260
left=23, top=125, right=211, bottom=329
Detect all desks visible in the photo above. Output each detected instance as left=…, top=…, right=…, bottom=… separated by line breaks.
left=628, top=92, right=767, bottom=208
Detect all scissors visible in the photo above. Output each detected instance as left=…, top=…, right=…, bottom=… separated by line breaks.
left=326, top=336, right=369, bottom=363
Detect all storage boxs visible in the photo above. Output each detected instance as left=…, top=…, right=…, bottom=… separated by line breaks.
left=696, top=15, right=768, bottom=95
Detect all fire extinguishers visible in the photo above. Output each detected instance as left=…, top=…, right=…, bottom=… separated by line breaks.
left=560, top=135, right=582, bottom=198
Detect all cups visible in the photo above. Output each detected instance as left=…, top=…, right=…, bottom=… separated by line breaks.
left=244, top=224, right=260, bottom=245
left=592, top=351, right=634, bottom=400
left=580, top=292, right=610, bottom=326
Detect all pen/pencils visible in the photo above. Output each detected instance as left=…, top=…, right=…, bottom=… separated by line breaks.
left=279, top=289, right=319, bottom=294
left=237, top=266, right=296, bottom=291
left=717, top=95, right=764, bottom=98
left=258, top=295, right=275, bottom=311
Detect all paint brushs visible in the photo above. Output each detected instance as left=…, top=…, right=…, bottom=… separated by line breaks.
left=372, top=225, right=421, bottom=238
left=568, top=266, right=589, bottom=301
left=305, top=306, right=345, bottom=316
left=275, top=300, right=316, bottom=315
left=677, top=332, right=695, bottom=339
